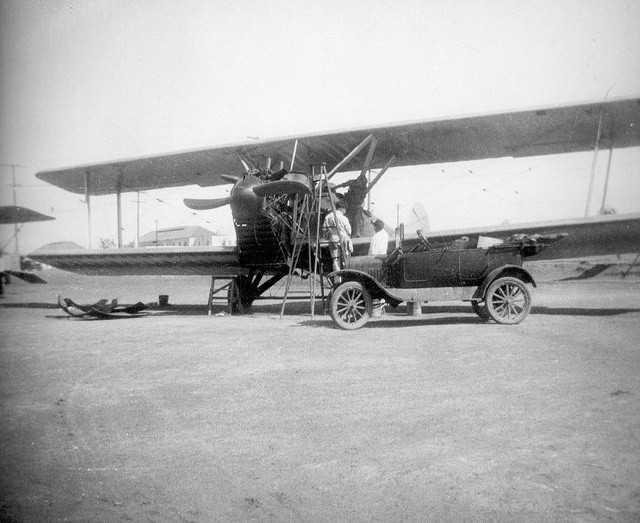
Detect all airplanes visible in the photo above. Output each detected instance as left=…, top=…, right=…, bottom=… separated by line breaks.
left=0, top=206, right=55, bottom=299
left=27, top=98, right=640, bottom=312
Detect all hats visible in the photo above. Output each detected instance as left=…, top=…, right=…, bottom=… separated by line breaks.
left=371, top=219, right=384, bottom=228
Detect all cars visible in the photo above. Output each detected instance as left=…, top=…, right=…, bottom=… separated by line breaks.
left=322, top=232, right=567, bottom=331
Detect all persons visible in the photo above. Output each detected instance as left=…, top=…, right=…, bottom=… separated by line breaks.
left=331, top=175, right=367, bottom=238
left=369, top=220, right=389, bottom=256
left=323, top=201, right=353, bottom=285
